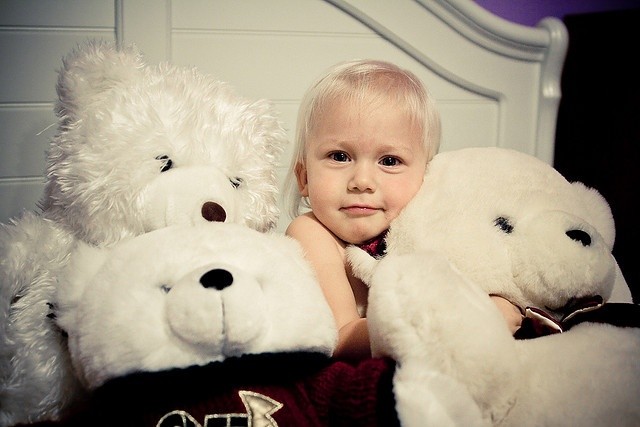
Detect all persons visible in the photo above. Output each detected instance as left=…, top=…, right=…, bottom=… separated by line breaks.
left=284, top=59, right=443, bottom=358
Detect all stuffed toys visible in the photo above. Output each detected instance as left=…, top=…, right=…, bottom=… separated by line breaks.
left=54, top=222, right=403, bottom=427
left=346, top=145, right=639, bottom=427
left=0, top=39, right=288, bottom=427
